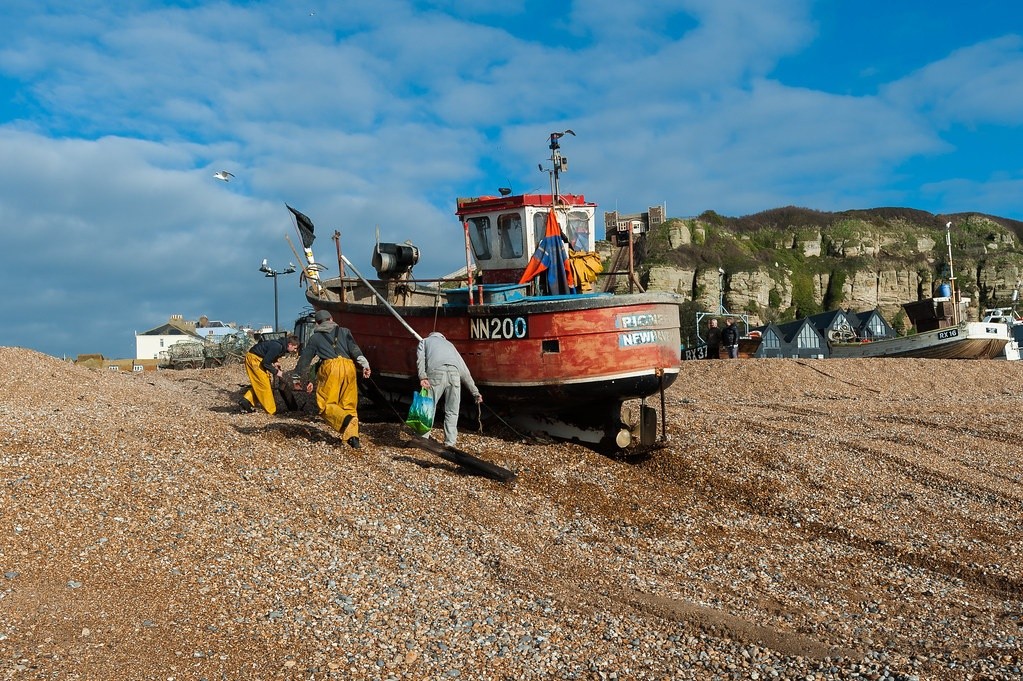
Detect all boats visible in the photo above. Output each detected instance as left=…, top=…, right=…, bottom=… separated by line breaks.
left=980, top=306, right=1023, bottom=352
left=829, top=221, right=1010, bottom=360
left=284, top=128, right=681, bottom=429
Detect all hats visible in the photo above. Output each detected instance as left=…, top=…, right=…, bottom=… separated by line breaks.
left=315, top=310, right=331, bottom=322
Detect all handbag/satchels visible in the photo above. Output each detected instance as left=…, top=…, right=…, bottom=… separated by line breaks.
left=405, top=386, right=435, bottom=433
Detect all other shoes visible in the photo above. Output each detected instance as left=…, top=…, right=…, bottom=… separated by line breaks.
left=412, top=437, right=428, bottom=446
left=342, top=415, right=358, bottom=440
left=444, top=443, right=454, bottom=450
left=347, top=436, right=359, bottom=449
left=273, top=408, right=284, bottom=415
left=238, top=397, right=256, bottom=413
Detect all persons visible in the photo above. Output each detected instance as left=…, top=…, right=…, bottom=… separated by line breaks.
left=296, top=310, right=372, bottom=448
left=722, top=318, right=738, bottom=358
left=406, top=332, right=483, bottom=448
left=705, top=318, right=722, bottom=359
left=238, top=336, right=300, bottom=415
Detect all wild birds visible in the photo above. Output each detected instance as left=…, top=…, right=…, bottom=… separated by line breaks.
left=213, top=170, right=235, bottom=182
left=546, top=129, right=576, bottom=142
